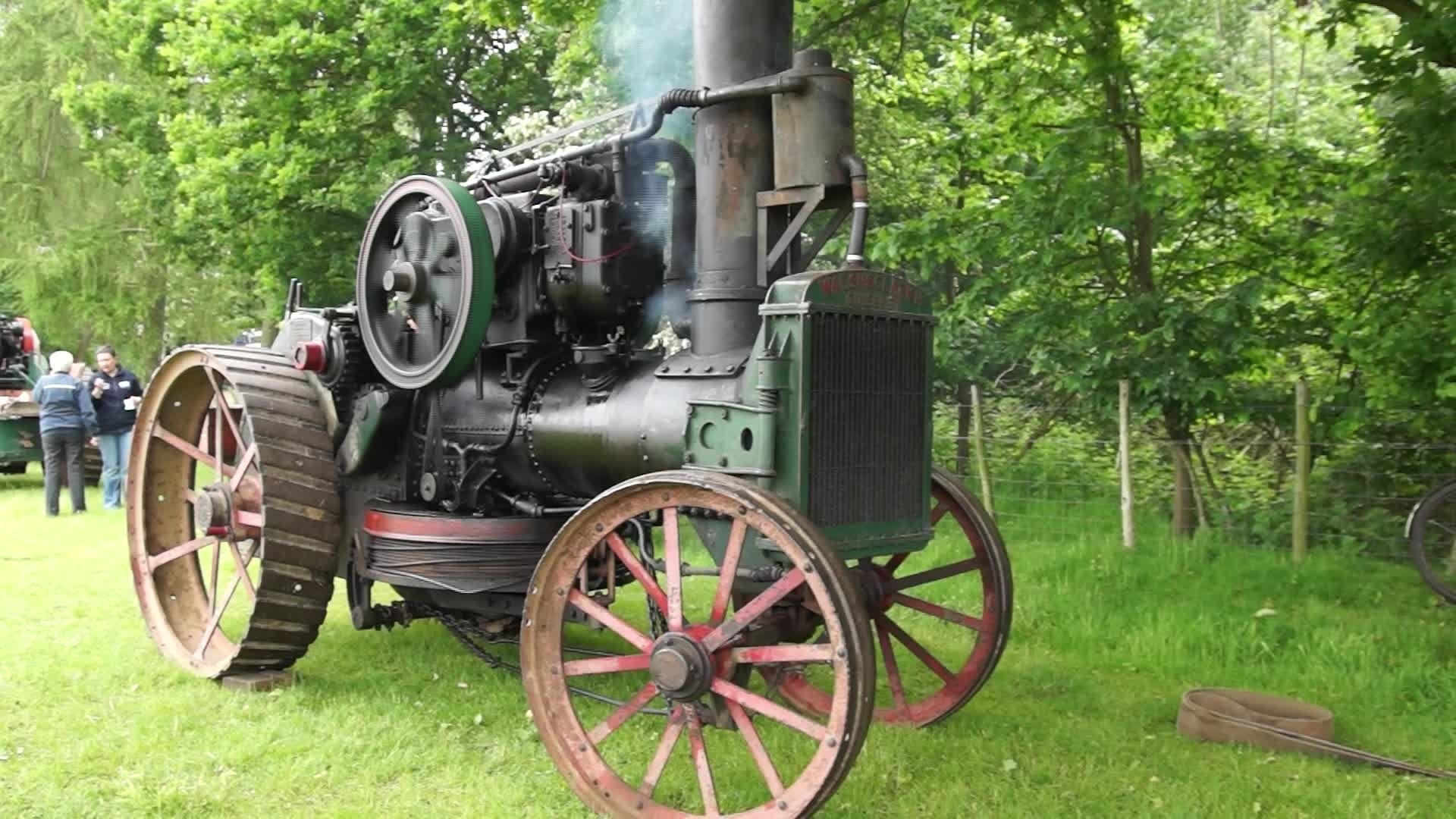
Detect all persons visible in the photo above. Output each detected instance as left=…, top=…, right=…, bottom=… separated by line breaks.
left=34, top=349, right=100, bottom=516
left=86, top=345, right=143, bottom=510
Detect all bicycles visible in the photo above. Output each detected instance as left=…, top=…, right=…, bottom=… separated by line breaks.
left=1404, top=478, right=1456, bottom=603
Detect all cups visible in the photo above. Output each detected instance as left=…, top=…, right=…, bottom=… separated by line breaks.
left=123, top=399, right=135, bottom=410
